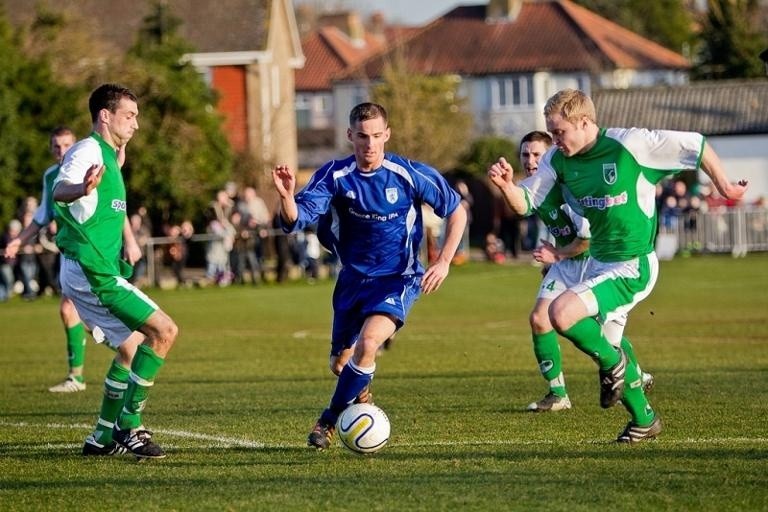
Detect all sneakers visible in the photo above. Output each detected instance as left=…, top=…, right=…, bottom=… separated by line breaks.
left=616, top=415, right=661, bottom=443
left=308, top=420, right=336, bottom=449
left=49, top=375, right=87, bottom=393
left=82, top=436, right=133, bottom=457
left=112, top=419, right=166, bottom=460
left=641, top=372, right=655, bottom=395
left=599, top=345, right=628, bottom=408
left=526, top=391, right=571, bottom=411
left=355, top=381, right=375, bottom=404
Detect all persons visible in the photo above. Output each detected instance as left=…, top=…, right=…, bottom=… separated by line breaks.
left=422, top=182, right=521, bottom=262
left=51, top=82, right=179, bottom=459
left=271, top=102, right=467, bottom=451
left=1, top=182, right=319, bottom=299
left=515, top=130, right=654, bottom=411
left=487, top=87, right=749, bottom=445
left=650, top=179, right=738, bottom=259
left=4, top=126, right=142, bottom=394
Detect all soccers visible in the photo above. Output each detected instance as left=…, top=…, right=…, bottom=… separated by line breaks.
left=337, top=404, right=392, bottom=455
